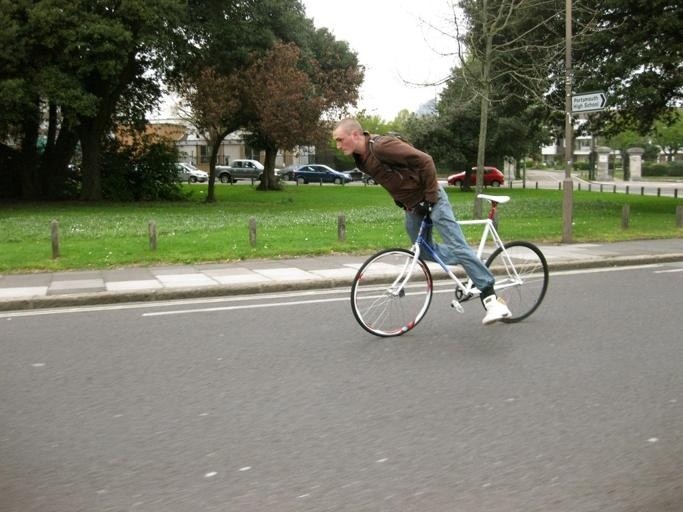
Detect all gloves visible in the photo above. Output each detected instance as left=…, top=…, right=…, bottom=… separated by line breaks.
left=412, top=201, right=432, bottom=220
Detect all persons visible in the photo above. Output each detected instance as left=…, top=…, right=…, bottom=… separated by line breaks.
left=328, top=117, right=513, bottom=325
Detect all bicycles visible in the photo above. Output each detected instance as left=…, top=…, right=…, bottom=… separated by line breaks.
left=349, top=193, right=551, bottom=337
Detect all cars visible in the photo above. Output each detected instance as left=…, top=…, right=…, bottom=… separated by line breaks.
left=175, top=163, right=208, bottom=183
left=275, top=165, right=299, bottom=181
left=292, top=164, right=352, bottom=187
left=447, top=167, right=504, bottom=189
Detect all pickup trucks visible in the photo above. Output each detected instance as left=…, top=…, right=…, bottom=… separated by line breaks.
left=214, top=159, right=276, bottom=184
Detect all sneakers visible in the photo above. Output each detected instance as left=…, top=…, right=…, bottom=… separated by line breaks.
left=481, top=294, right=513, bottom=325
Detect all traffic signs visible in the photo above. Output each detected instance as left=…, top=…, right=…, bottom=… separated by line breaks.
left=571, top=90, right=608, bottom=115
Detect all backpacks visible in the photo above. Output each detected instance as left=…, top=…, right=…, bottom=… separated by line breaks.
left=369, top=130, right=415, bottom=179
